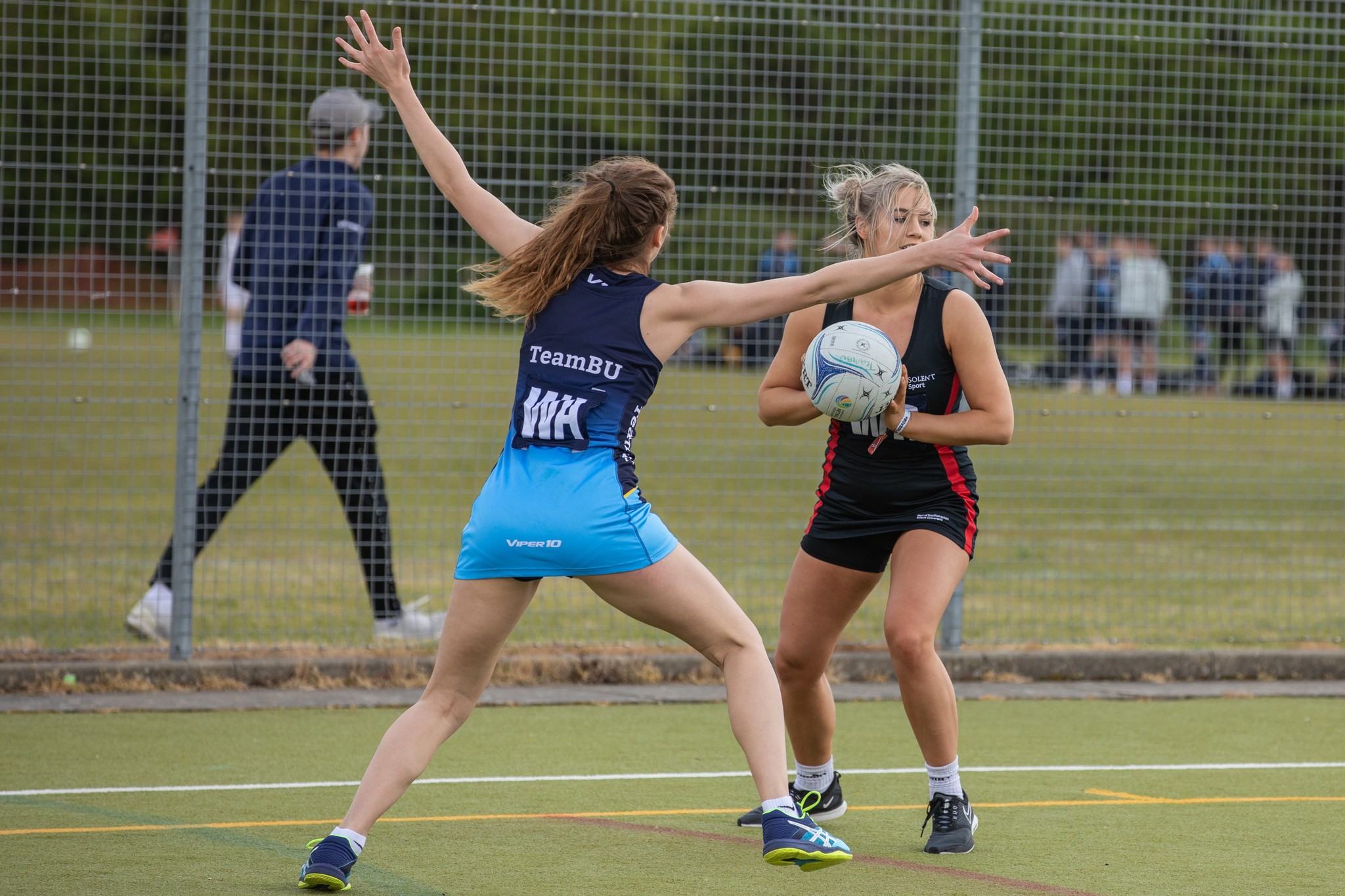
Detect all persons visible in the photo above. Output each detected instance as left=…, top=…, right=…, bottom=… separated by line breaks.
left=124, top=85, right=449, bottom=646
left=295, top=11, right=1011, bottom=888
left=736, top=158, right=1015, bottom=856
left=753, top=229, right=1305, bottom=402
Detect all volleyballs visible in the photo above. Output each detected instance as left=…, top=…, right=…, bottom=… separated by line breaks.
left=801, top=320, right=903, bottom=423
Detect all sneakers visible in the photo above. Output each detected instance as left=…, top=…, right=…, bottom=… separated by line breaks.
left=373, top=597, right=444, bottom=642
left=737, top=771, right=847, bottom=827
left=124, top=582, right=171, bottom=641
left=298, top=835, right=358, bottom=890
left=762, top=791, right=852, bottom=872
left=920, top=788, right=979, bottom=853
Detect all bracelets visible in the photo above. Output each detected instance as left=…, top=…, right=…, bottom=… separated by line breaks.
left=867, top=408, right=911, bottom=456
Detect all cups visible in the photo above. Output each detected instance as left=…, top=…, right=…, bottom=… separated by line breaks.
left=347, top=264, right=374, bottom=316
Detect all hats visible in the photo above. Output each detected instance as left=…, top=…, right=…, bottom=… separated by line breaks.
left=307, top=86, right=382, bottom=135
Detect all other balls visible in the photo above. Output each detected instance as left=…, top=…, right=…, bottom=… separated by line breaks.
left=69, top=330, right=94, bottom=352
